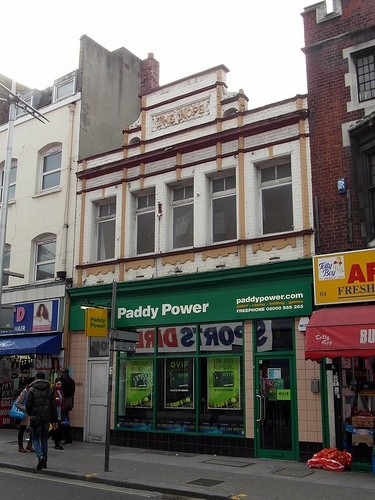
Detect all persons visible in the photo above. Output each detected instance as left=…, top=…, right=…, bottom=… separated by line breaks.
left=61, top=370, right=75, bottom=444
left=10, top=382, right=32, bottom=452
left=53, top=378, right=65, bottom=450
left=25, top=371, right=58, bottom=471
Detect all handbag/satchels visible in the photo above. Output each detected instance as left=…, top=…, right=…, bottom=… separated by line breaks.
left=9, top=399, right=27, bottom=420
left=56, top=406, right=61, bottom=420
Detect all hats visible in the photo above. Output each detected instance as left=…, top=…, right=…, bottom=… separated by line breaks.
left=61, top=368, right=69, bottom=374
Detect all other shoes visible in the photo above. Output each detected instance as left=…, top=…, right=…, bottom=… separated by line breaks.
left=62, top=442, right=71, bottom=445
left=55, top=445, right=64, bottom=450
left=37, top=456, right=47, bottom=470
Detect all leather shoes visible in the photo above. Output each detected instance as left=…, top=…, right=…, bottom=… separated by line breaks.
left=26, top=444, right=36, bottom=452
left=18, top=445, right=27, bottom=453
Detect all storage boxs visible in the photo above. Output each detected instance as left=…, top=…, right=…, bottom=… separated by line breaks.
left=352, top=416, right=375, bottom=427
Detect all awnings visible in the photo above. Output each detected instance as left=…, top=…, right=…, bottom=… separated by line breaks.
left=1, top=335, right=61, bottom=357
left=305, top=303, right=375, bottom=360
